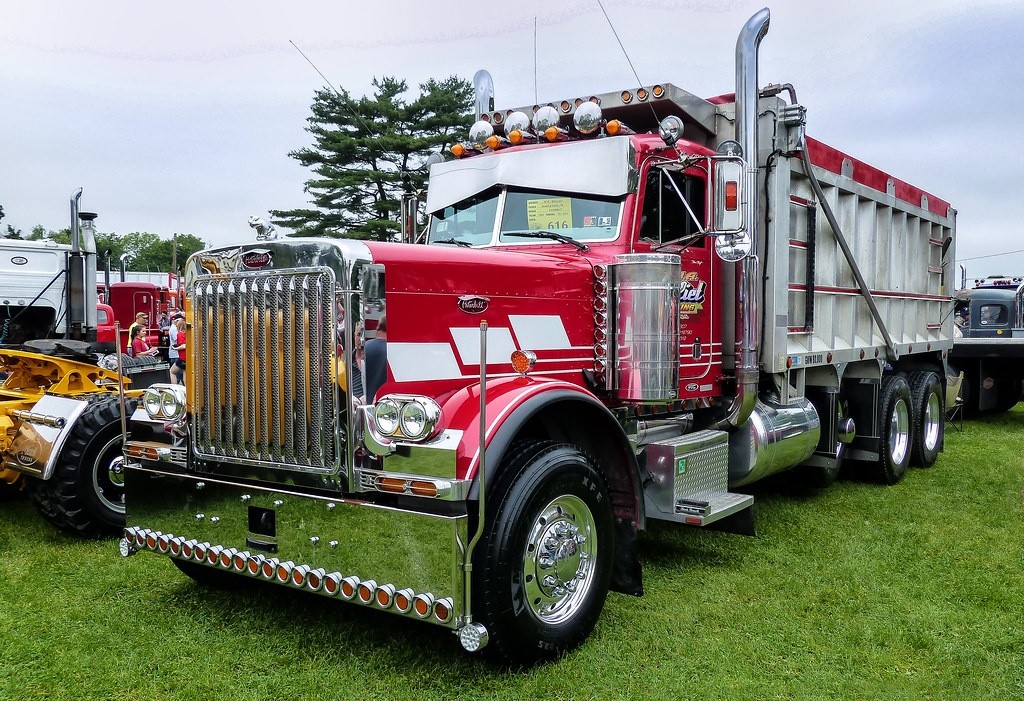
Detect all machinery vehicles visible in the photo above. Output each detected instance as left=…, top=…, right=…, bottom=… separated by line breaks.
left=955, top=263, right=1024, bottom=338
left=0, top=235, right=185, bottom=359
left=0, top=187, right=198, bottom=538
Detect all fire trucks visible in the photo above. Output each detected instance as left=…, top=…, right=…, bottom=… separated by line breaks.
left=115, top=8, right=962, bottom=668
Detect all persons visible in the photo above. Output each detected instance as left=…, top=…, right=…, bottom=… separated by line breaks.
left=353, top=315, right=387, bottom=410
left=126, top=310, right=186, bottom=385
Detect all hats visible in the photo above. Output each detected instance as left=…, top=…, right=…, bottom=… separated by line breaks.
left=172, top=314, right=185, bottom=320
left=136, top=311, right=148, bottom=318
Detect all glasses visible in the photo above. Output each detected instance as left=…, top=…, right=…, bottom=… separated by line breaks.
left=141, top=317, right=147, bottom=319
